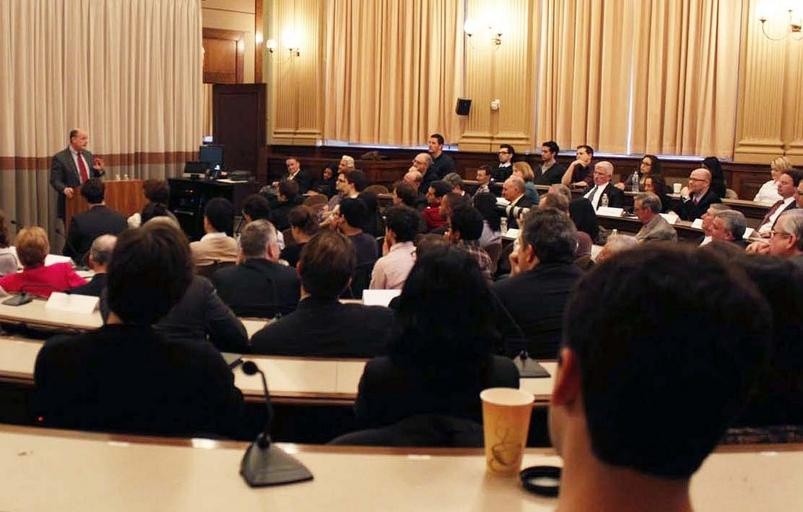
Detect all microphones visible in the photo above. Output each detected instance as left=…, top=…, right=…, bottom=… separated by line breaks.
left=490, top=289, right=551, bottom=378
left=241, top=360, right=313, bottom=488
left=54, top=228, right=89, bottom=271
left=11, top=220, right=23, bottom=229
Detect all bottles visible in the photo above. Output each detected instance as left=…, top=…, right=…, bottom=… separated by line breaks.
left=610, top=228, right=618, bottom=235
left=498, top=216, right=508, bottom=235
left=602, top=193, right=608, bottom=207
left=632, top=171, right=640, bottom=193
left=114, top=173, right=128, bottom=180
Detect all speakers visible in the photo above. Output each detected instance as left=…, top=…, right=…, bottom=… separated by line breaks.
left=456, top=98, right=472, bottom=115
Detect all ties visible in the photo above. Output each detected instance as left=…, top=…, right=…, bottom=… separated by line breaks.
left=589, top=187, right=598, bottom=202
left=761, top=200, right=784, bottom=225
left=78, top=152, right=88, bottom=184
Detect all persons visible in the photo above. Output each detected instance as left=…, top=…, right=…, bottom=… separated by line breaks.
left=144, top=216, right=248, bottom=353
left=495, top=206, right=585, bottom=360
left=36, top=223, right=248, bottom=440
left=249, top=232, right=375, bottom=358
left=353, top=246, right=520, bottom=428
left=545, top=243, right=770, bottom=512
left=1, top=129, right=802, bottom=320
left=730, top=255, right=801, bottom=426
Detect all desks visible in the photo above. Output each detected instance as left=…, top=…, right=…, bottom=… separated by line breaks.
left=0, top=289, right=273, bottom=348
left=0, top=332, right=558, bottom=408
left=343, top=289, right=402, bottom=308
left=457, top=173, right=776, bottom=264
left=1, top=423, right=803, bottom=512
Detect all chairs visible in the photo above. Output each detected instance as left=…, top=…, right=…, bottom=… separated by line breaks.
left=302, top=193, right=329, bottom=213
left=376, top=235, right=384, bottom=257
left=326, top=416, right=484, bottom=447
left=229, top=304, right=297, bottom=319
left=197, top=260, right=235, bottom=277
left=282, top=227, right=295, bottom=246
left=362, top=184, right=389, bottom=194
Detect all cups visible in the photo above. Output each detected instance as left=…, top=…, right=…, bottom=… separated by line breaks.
left=673, top=182, right=682, bottom=193
left=478, top=386, right=536, bottom=476
